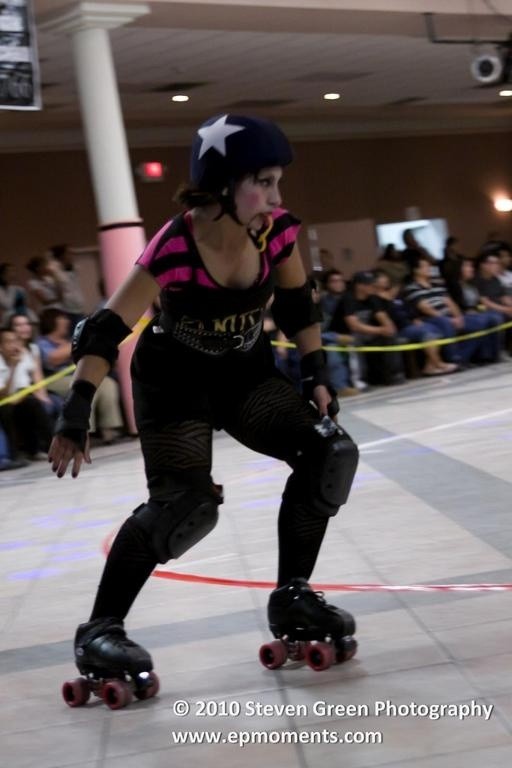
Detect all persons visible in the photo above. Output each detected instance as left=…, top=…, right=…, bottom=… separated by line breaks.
left=0, top=242, right=125, bottom=471
left=45, top=111, right=359, bottom=710
left=262, top=227, right=512, bottom=396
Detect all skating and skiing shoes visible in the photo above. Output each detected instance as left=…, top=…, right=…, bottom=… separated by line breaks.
left=63, top=618, right=159, bottom=708
left=260, top=578, right=355, bottom=671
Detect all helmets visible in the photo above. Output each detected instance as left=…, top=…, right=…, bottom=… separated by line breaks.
left=189, top=114, right=293, bottom=205
